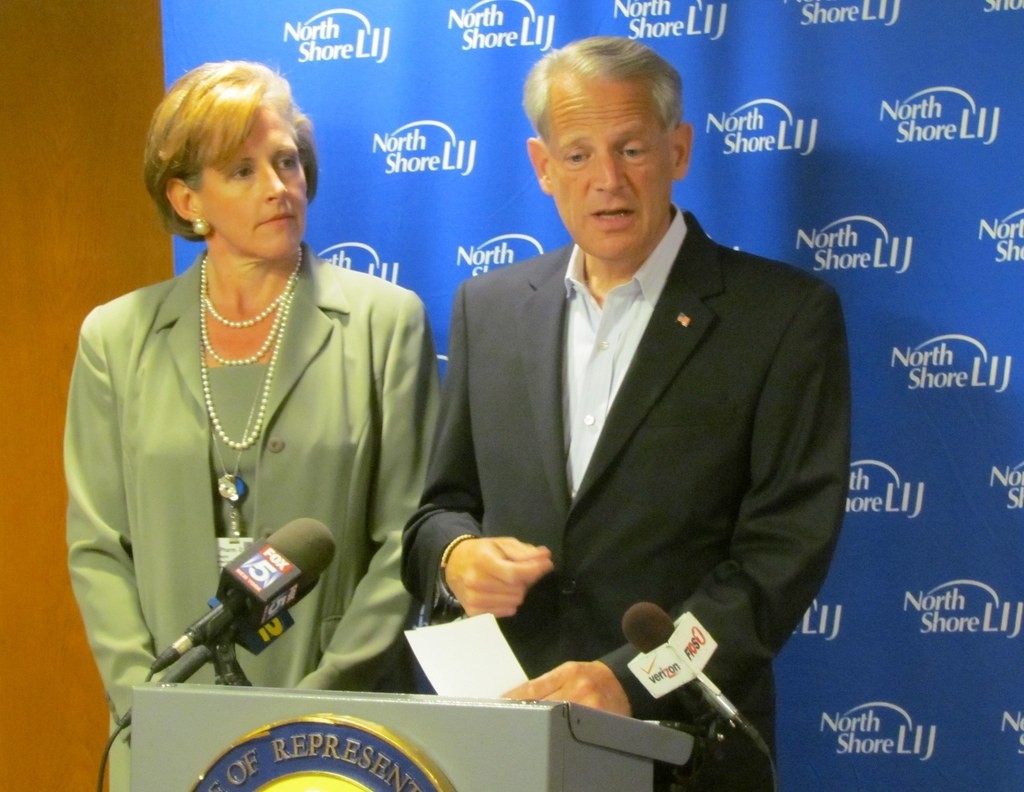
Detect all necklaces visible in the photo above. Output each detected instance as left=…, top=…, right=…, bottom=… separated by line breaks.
left=198, top=249, right=304, bottom=539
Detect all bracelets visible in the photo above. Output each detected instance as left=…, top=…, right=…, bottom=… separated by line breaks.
left=439, top=534, right=475, bottom=569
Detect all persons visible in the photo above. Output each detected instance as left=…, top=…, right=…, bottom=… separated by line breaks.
left=64, top=58, right=441, bottom=792
left=397, top=37, right=853, bottom=792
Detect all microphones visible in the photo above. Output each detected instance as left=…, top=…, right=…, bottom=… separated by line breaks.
left=623, top=602, right=772, bottom=755
left=121, top=517, right=336, bottom=728
